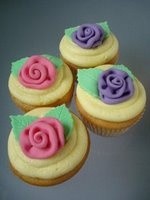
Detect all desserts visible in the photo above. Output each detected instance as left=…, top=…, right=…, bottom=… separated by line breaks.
left=75, top=64, right=146, bottom=138
left=8, top=54, right=74, bottom=113
left=8, top=102, right=90, bottom=187
left=59, top=20, right=119, bottom=83
